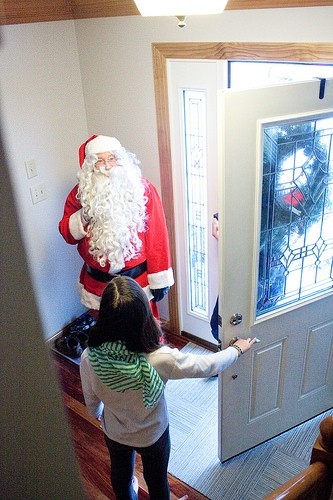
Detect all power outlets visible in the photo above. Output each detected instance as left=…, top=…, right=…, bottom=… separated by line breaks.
left=31, top=181, right=48, bottom=205
left=25, top=160, right=38, bottom=179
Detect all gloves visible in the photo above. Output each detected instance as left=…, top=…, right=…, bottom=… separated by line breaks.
left=150, top=286, right=170, bottom=301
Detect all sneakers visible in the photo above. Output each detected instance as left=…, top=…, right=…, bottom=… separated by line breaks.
left=131, top=476, right=140, bottom=494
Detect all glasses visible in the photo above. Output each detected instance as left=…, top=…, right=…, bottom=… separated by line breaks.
left=95, top=157, right=117, bottom=167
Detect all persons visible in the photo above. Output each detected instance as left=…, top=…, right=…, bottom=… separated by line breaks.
left=58, top=134, right=175, bottom=344
left=80, top=276, right=257, bottom=500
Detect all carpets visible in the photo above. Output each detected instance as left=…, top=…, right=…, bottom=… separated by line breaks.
left=164, top=342, right=333, bottom=500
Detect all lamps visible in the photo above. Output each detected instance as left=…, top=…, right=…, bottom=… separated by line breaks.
left=133, top=0, right=228, bottom=28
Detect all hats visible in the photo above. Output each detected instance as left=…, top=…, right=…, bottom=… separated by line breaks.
left=79, top=135, right=122, bottom=169
